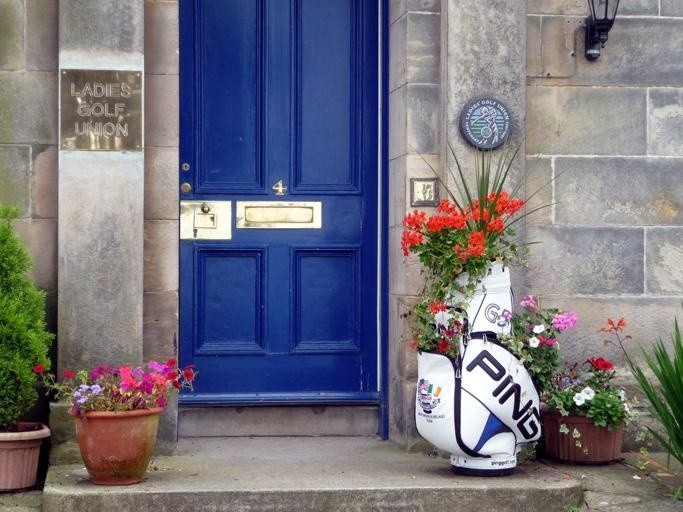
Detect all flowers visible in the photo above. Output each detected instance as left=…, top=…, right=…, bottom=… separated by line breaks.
left=505, top=291, right=633, bottom=423
left=387, top=146, right=532, bottom=354
left=33, top=358, right=196, bottom=412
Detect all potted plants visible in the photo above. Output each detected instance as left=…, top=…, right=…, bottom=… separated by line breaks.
left=1, top=202, right=57, bottom=495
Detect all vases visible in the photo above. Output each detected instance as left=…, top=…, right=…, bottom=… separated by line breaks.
left=414, top=259, right=542, bottom=468
left=67, top=406, right=161, bottom=485
left=538, top=409, right=626, bottom=463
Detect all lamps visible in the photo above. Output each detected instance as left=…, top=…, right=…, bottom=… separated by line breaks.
left=576, top=1, right=618, bottom=63
